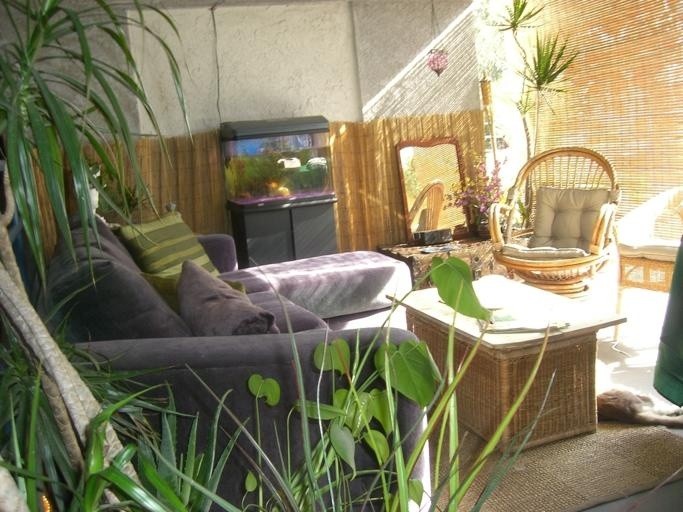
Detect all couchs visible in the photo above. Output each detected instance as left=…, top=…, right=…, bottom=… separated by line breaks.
left=34, top=211, right=435, bottom=512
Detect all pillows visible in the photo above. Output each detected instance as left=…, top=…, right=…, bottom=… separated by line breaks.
left=117, top=218, right=283, bottom=335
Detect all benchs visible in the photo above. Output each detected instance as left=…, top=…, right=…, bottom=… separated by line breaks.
left=237, top=250, right=413, bottom=329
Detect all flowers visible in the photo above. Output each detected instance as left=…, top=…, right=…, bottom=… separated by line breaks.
left=451, top=154, right=504, bottom=227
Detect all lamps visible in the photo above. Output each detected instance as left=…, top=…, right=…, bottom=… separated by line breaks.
left=428, top=0, right=448, bottom=78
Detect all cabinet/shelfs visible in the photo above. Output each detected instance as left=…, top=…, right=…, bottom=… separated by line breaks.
left=224, top=199, right=339, bottom=272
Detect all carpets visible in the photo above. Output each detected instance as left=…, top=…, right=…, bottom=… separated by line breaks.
left=423, top=404, right=681, bottom=512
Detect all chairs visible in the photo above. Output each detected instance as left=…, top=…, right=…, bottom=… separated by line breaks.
left=409, top=179, right=446, bottom=231
left=488, top=146, right=683, bottom=342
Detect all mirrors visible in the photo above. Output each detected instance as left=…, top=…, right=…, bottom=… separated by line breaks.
left=395, top=136, right=473, bottom=244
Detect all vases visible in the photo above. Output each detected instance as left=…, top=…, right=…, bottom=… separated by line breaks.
left=469, top=223, right=491, bottom=241
